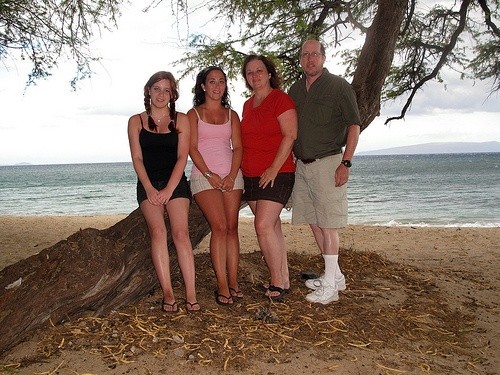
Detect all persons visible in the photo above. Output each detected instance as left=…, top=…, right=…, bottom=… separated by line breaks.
left=240, top=54, right=297, bottom=298
left=186, top=66, right=244, bottom=305
left=127, top=71, right=201, bottom=311
left=287, top=39, right=360, bottom=305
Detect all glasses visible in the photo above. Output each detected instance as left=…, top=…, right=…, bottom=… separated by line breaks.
left=300, top=51, right=325, bottom=58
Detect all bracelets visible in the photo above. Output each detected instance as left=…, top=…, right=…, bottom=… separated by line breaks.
left=204, top=170, right=213, bottom=179
left=228, top=174, right=235, bottom=183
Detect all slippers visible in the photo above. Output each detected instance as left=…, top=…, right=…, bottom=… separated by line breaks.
left=228, top=286, right=243, bottom=299
left=162, top=298, right=179, bottom=313
left=185, top=299, right=201, bottom=313
left=214, top=290, right=234, bottom=307
left=261, top=282, right=290, bottom=293
left=261, top=285, right=285, bottom=301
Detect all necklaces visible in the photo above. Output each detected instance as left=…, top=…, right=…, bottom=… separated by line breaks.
left=151, top=111, right=169, bottom=123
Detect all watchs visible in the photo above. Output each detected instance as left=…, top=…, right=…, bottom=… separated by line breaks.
left=341, top=159, right=352, bottom=168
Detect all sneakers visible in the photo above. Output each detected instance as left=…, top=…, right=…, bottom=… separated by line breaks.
left=304, top=273, right=347, bottom=291
left=306, top=281, right=339, bottom=305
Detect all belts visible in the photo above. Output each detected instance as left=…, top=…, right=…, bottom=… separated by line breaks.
left=300, top=159, right=315, bottom=164
